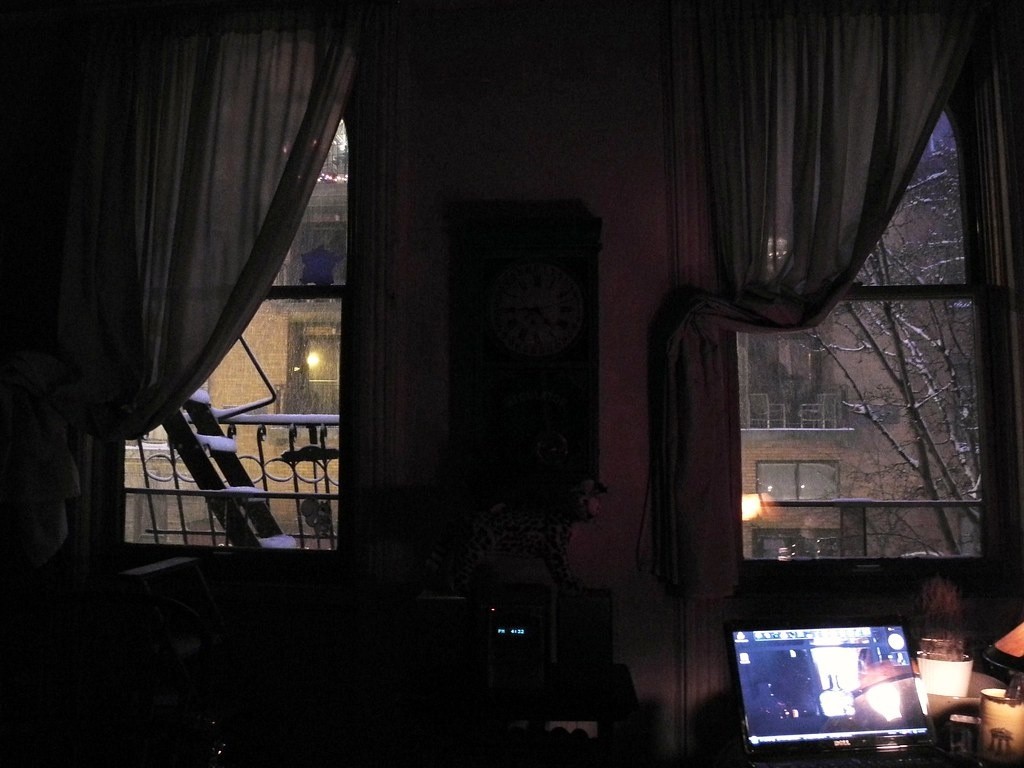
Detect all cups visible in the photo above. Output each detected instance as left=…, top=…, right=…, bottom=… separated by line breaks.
left=978, top=688, right=1024, bottom=764
left=949, top=713, right=982, bottom=756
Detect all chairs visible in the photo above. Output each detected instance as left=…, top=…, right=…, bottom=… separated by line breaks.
left=799, top=393, right=837, bottom=429
left=750, top=393, right=787, bottom=428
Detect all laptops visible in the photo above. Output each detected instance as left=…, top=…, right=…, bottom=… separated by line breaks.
left=724, top=613, right=979, bottom=768
